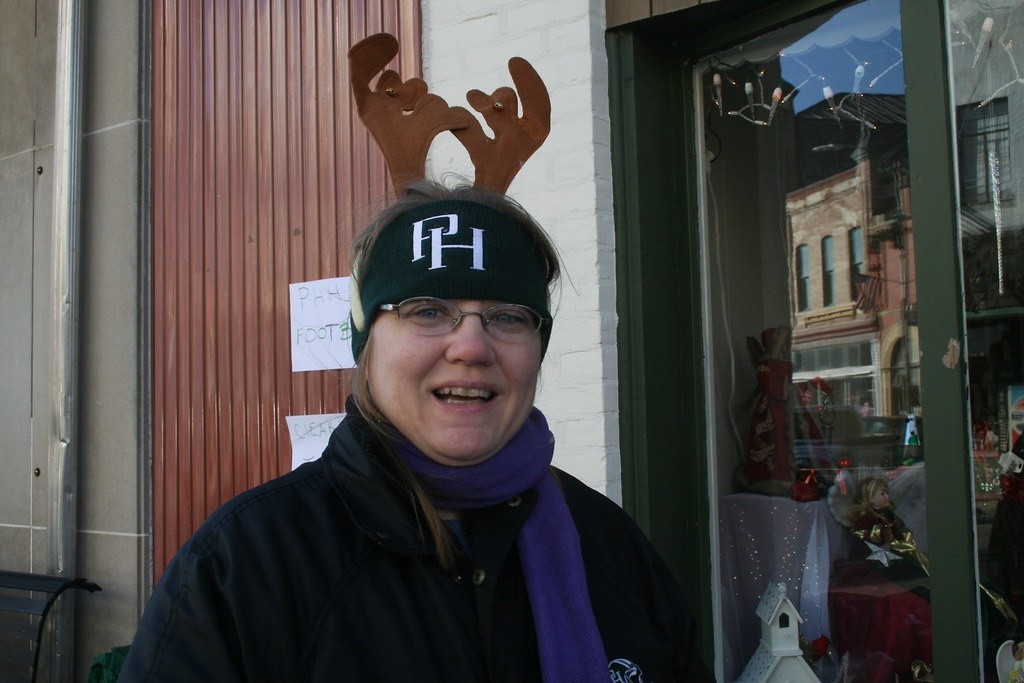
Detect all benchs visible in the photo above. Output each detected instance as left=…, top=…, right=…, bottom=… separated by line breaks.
left=0, top=569, right=103, bottom=683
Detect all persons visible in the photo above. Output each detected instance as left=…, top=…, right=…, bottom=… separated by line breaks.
left=739, top=325, right=841, bottom=494
left=987, top=431, right=1024, bottom=650
left=118, top=178, right=704, bottom=683
left=849, top=477, right=929, bottom=594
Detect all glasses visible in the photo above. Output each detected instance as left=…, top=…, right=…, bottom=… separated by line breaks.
left=380, top=296, right=547, bottom=344
